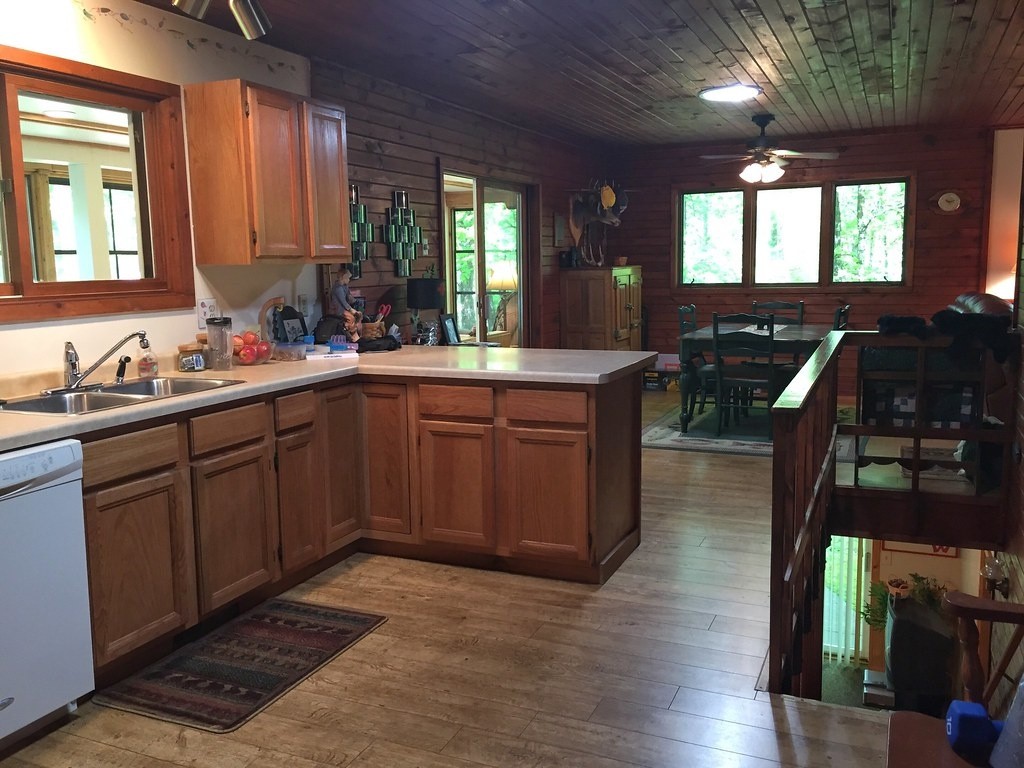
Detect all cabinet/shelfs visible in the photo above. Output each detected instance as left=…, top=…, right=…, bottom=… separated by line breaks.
left=84, top=381, right=360, bottom=663
left=360, top=370, right=641, bottom=586
left=184, top=79, right=353, bottom=266
left=560, top=267, right=642, bottom=351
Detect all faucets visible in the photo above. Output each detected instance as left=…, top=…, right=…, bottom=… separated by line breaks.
left=112, top=355, right=132, bottom=385
left=63, top=330, right=150, bottom=390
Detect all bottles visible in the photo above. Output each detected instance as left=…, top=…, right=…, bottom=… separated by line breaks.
left=304, top=336, right=315, bottom=352
left=137, top=346, right=158, bottom=377
left=178, top=333, right=213, bottom=372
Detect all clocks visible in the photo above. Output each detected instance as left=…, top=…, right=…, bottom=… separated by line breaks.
left=937, top=192, right=961, bottom=212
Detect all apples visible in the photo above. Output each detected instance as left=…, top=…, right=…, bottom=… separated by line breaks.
left=232, top=330, right=272, bottom=364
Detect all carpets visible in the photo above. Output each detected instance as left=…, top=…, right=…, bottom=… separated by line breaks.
left=642, top=398, right=870, bottom=465
left=91, top=598, right=387, bottom=735
left=901, top=446, right=966, bottom=481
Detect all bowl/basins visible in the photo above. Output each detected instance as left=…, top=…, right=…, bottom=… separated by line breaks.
left=274, top=342, right=307, bottom=361
left=232, top=343, right=276, bottom=365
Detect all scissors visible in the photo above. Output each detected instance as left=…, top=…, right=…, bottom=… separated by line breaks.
left=375, top=303, right=392, bottom=321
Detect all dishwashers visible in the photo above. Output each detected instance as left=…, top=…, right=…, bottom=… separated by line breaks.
left=0, top=439, right=95, bottom=739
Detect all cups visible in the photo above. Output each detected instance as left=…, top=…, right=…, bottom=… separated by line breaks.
left=206, top=318, right=232, bottom=371
left=362, top=321, right=386, bottom=340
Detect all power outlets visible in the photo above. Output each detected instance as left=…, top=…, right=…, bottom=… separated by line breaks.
left=299, top=294, right=308, bottom=315
left=199, top=299, right=219, bottom=328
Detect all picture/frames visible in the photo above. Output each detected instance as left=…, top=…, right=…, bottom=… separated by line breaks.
left=278, top=313, right=307, bottom=342
left=439, top=314, right=461, bottom=344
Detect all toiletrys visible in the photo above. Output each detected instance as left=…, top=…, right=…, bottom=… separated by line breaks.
left=137, top=346, right=159, bottom=378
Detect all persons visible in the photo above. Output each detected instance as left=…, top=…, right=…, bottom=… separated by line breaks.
left=329, top=268, right=361, bottom=340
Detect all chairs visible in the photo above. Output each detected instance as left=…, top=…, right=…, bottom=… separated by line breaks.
left=677, top=301, right=852, bottom=439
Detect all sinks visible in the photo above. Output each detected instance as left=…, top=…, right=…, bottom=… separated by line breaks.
left=74, top=376, right=247, bottom=398
left=0, top=392, right=149, bottom=417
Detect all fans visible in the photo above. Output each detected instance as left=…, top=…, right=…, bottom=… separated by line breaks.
left=702, top=114, right=839, bottom=164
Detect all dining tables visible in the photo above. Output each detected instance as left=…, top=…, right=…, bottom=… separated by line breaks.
left=679, top=320, right=847, bottom=433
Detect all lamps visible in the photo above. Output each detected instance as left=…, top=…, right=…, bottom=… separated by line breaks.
left=986, top=562, right=1009, bottom=598
left=739, top=158, right=786, bottom=184
left=408, top=279, right=446, bottom=345
